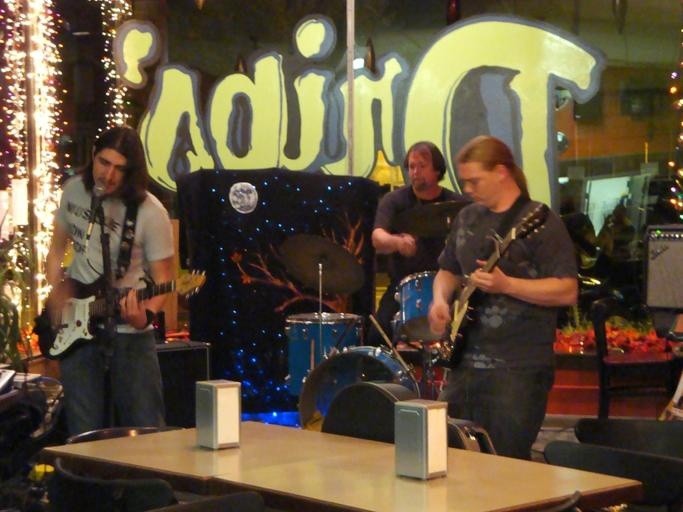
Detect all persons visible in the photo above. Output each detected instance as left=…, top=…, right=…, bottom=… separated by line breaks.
left=371, top=141, right=471, bottom=353
left=48, top=128, right=174, bottom=501
left=429, top=135, right=579, bottom=461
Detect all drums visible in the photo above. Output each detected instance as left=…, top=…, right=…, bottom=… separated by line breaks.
left=298, top=345, right=421, bottom=432
left=283, top=310, right=364, bottom=397
left=393, top=269, right=460, bottom=343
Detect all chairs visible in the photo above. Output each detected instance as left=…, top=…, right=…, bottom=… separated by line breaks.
left=55, top=424, right=265, bottom=511
left=545, top=414, right=683, bottom=510
left=589, top=293, right=683, bottom=418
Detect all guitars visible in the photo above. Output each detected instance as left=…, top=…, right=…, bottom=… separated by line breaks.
left=37, top=268, right=207, bottom=361
left=429, top=202, right=550, bottom=363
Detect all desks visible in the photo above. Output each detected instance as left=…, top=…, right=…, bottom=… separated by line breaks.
left=43, top=419, right=646, bottom=512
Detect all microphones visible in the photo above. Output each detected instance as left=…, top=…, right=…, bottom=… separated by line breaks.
left=86, top=183, right=107, bottom=240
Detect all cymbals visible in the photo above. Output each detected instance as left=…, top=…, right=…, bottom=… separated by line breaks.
left=278, top=231, right=368, bottom=296
left=395, top=198, right=473, bottom=237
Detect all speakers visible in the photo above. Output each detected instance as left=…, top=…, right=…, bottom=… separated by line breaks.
left=158, top=342, right=209, bottom=429
left=394, top=399, right=447, bottom=480
left=196, top=380, right=241, bottom=450
